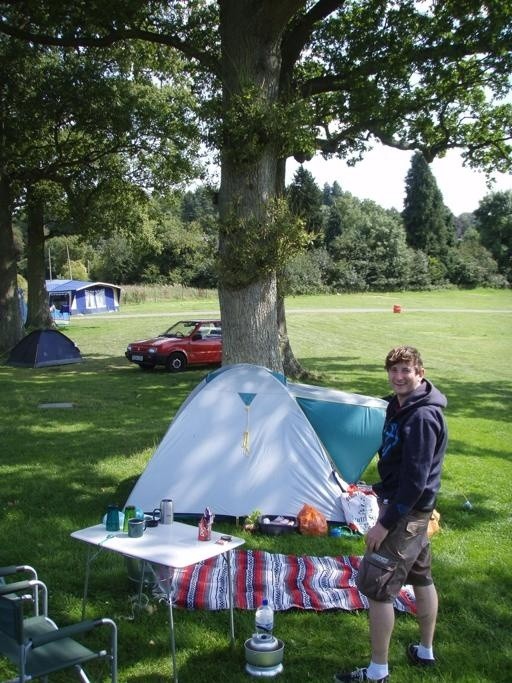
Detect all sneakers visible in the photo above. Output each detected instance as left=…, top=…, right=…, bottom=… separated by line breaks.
left=333, top=666, right=389, bottom=683
left=408, top=642, right=435, bottom=667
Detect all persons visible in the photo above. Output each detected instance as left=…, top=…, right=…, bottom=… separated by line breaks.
left=332, top=343, right=449, bottom=682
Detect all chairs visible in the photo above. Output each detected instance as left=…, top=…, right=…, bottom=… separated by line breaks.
left=0, top=560, right=40, bottom=682
left=0, top=594, right=119, bottom=681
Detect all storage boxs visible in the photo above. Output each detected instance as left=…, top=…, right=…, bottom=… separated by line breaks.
left=258, top=512, right=301, bottom=537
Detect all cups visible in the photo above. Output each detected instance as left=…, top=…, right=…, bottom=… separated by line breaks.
left=128, top=518, right=147, bottom=538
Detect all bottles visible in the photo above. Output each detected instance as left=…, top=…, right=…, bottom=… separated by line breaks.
left=137, top=507, right=146, bottom=532
left=329, top=526, right=342, bottom=536
left=254, top=599, right=273, bottom=642
left=105, top=503, right=120, bottom=532
left=123, top=505, right=137, bottom=532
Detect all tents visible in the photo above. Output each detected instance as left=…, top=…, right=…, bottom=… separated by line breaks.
left=120, top=360, right=391, bottom=524
left=5, top=327, right=87, bottom=371
left=49, top=277, right=122, bottom=314
left=43, top=278, right=73, bottom=296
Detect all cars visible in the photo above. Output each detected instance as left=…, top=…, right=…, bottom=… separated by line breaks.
left=125, top=318, right=222, bottom=370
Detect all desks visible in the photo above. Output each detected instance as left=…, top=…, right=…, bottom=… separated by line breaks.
left=70, top=514, right=245, bottom=681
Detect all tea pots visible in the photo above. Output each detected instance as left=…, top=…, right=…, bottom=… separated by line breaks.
left=153, top=498, right=174, bottom=525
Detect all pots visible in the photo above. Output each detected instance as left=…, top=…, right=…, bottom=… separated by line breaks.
left=243, top=637, right=285, bottom=667
